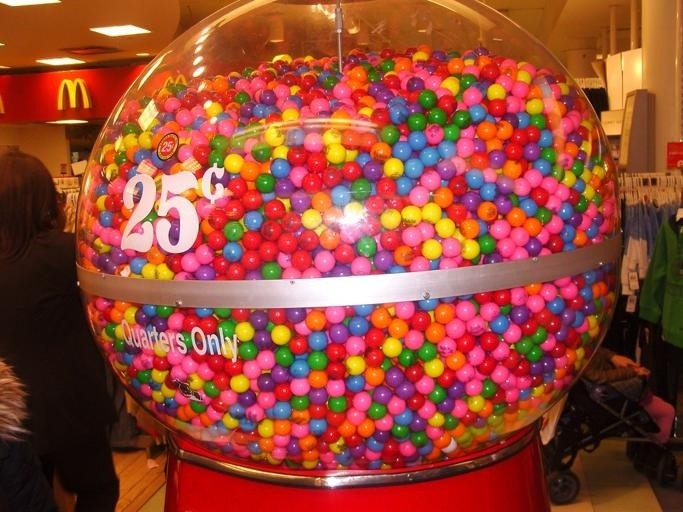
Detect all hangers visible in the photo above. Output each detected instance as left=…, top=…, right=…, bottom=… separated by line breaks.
left=618, top=172, right=683, bottom=207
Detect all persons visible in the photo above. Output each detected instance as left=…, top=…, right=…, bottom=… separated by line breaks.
left=582, top=348, right=676, bottom=444
left=1, top=358, right=61, bottom=512
left=0, top=151, right=153, bottom=512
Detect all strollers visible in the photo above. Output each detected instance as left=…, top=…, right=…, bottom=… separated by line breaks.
left=542, top=344, right=681, bottom=503
